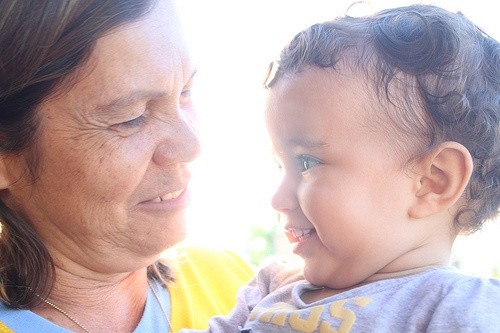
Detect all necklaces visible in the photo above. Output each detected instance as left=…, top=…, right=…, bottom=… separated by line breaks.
left=5, top=279, right=98, bottom=331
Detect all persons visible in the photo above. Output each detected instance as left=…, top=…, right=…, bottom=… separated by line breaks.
left=0, top=1, right=262, bottom=332
left=180, top=1, right=500, bottom=332
